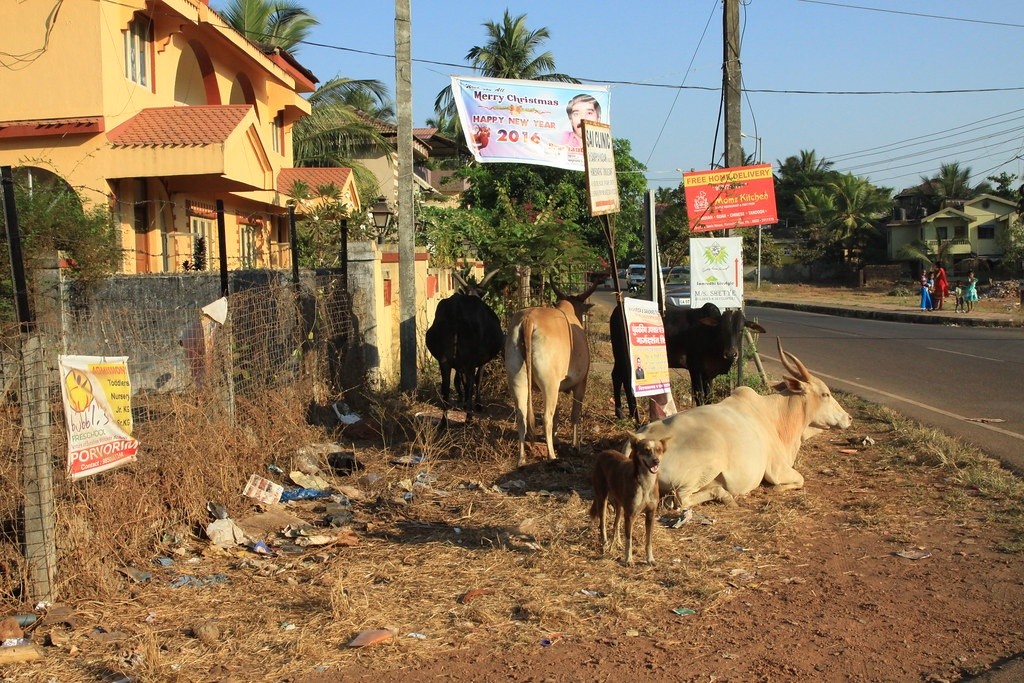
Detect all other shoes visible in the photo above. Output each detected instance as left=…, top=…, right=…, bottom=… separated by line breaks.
left=955, top=310, right=959, bottom=313
left=962, top=309, right=966, bottom=313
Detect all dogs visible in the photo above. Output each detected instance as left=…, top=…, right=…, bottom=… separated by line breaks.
left=586, top=429, right=673, bottom=568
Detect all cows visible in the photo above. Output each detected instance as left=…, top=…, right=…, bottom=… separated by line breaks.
left=504, top=273, right=607, bottom=469
left=620, top=335, right=854, bottom=508
left=425, top=268, right=504, bottom=414
left=609, top=299, right=767, bottom=428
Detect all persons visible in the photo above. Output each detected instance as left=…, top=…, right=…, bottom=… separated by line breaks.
left=955, top=269, right=978, bottom=313
left=921, top=267, right=949, bottom=311
left=635, top=357, right=644, bottom=379
left=558, top=95, right=602, bottom=150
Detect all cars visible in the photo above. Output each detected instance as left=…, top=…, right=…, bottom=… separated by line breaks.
left=625, top=264, right=691, bottom=311
